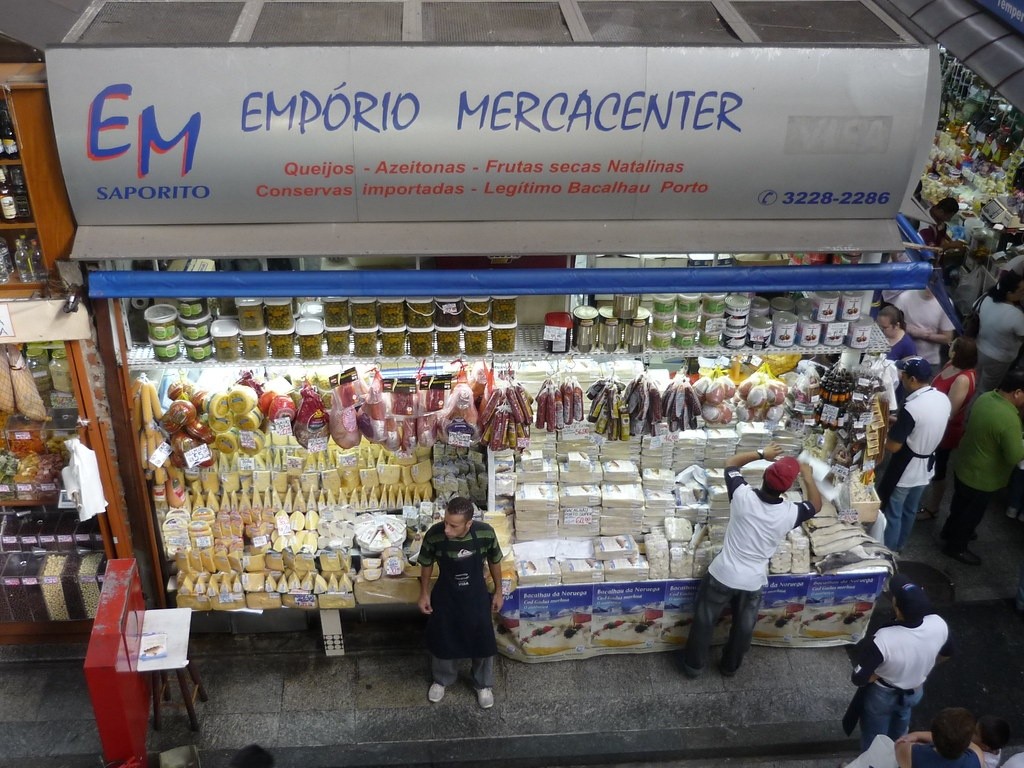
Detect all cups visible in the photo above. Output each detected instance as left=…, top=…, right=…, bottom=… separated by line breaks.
left=144, top=304, right=181, bottom=363
left=488, top=295, right=519, bottom=354
left=323, top=297, right=353, bottom=355
left=177, top=297, right=213, bottom=362
left=406, top=296, right=435, bottom=357
left=348, top=297, right=378, bottom=358
left=235, top=297, right=267, bottom=360
left=462, top=295, right=491, bottom=356
left=649, top=294, right=727, bottom=351
left=378, top=296, right=407, bottom=357
left=264, top=297, right=302, bottom=359
left=295, top=301, right=325, bottom=359
left=210, top=297, right=241, bottom=361
left=434, top=296, right=462, bottom=357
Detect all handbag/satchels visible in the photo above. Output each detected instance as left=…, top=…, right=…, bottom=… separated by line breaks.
left=962, top=293, right=990, bottom=335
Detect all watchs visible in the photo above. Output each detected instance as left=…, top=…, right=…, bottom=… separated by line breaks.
left=756, top=448, right=764, bottom=460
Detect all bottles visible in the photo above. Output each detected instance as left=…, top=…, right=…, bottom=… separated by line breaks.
left=0, top=236, right=15, bottom=283
left=813, top=367, right=854, bottom=431
left=543, top=312, right=573, bottom=353
left=0, top=101, right=20, bottom=160
left=0, top=165, right=32, bottom=221
left=15, top=233, right=45, bottom=283
left=49, top=349, right=73, bottom=392
left=26, top=349, right=53, bottom=393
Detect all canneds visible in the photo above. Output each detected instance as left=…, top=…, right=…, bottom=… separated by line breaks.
left=724, top=291, right=872, bottom=349
left=572, top=294, right=650, bottom=352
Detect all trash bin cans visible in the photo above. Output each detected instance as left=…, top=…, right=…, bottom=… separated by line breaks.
left=865, top=560, right=955, bottom=646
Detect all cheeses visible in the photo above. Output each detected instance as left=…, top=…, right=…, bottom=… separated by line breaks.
left=152, top=431, right=517, bottom=611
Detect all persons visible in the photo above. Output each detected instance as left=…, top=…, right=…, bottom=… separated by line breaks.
left=963, top=271, right=1024, bottom=421
left=839, top=573, right=949, bottom=768
left=869, top=165, right=1024, bottom=568
left=894, top=705, right=1024, bottom=768
left=670, top=441, right=823, bottom=679
left=876, top=357, right=951, bottom=553
left=417, top=497, right=506, bottom=709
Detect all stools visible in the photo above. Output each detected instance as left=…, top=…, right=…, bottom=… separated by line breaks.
left=137, top=607, right=209, bottom=734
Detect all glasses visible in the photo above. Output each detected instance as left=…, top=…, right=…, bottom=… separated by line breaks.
left=877, top=322, right=898, bottom=330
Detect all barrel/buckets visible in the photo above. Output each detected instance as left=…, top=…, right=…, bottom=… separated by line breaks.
left=959, top=211, right=985, bottom=242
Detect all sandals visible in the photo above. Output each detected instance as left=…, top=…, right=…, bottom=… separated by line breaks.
left=915, top=506, right=939, bottom=520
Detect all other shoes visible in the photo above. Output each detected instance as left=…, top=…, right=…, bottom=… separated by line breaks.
left=965, top=532, right=980, bottom=541
left=947, top=545, right=980, bottom=567
left=475, top=683, right=493, bottom=709
left=671, top=652, right=698, bottom=678
left=428, top=680, right=446, bottom=702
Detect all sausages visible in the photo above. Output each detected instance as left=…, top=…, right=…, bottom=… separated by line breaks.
left=586, top=377, right=701, bottom=440
left=132, top=379, right=186, bottom=510
left=481, top=382, right=584, bottom=450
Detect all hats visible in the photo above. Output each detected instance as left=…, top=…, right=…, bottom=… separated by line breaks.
left=765, top=457, right=800, bottom=491
left=889, top=575, right=930, bottom=621
left=893, top=357, right=931, bottom=384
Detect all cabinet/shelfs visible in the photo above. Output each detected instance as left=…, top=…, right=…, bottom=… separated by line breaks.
left=0, top=82, right=135, bottom=647
